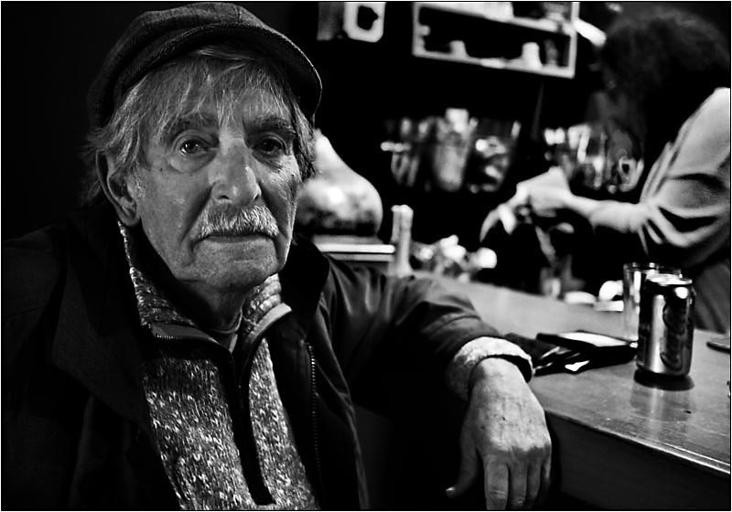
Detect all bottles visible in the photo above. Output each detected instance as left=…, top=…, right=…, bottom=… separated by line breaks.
left=382, top=203, right=414, bottom=281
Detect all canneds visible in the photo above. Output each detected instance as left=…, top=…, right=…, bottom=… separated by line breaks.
left=637, top=270, right=696, bottom=378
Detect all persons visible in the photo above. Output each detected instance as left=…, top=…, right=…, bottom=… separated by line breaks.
left=2, top=2, right=554, bottom=509
left=516, top=4, right=731, bottom=333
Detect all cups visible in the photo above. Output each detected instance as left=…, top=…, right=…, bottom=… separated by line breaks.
left=621, top=263, right=664, bottom=350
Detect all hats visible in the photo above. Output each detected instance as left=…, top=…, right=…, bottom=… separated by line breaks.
left=87, top=1, right=326, bottom=137
